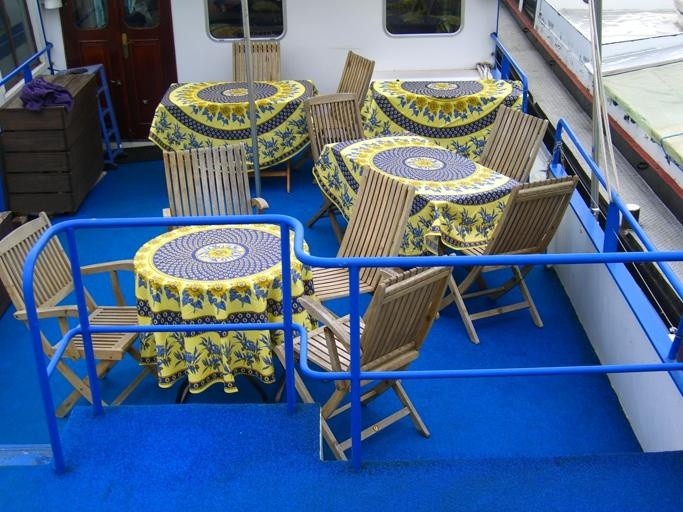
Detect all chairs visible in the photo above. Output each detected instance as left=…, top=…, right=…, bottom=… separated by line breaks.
left=0, top=212, right=161, bottom=418
left=318, top=49, right=376, bottom=130
left=311, top=165, right=415, bottom=303
left=435, top=173, right=579, bottom=344
left=232, top=39, right=280, bottom=83
left=303, top=92, right=364, bottom=246
left=274, top=251, right=456, bottom=462
left=163, top=142, right=269, bottom=231
left=479, top=104, right=549, bottom=183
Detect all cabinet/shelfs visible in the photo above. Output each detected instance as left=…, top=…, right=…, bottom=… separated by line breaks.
left=0, top=72, right=104, bottom=215
